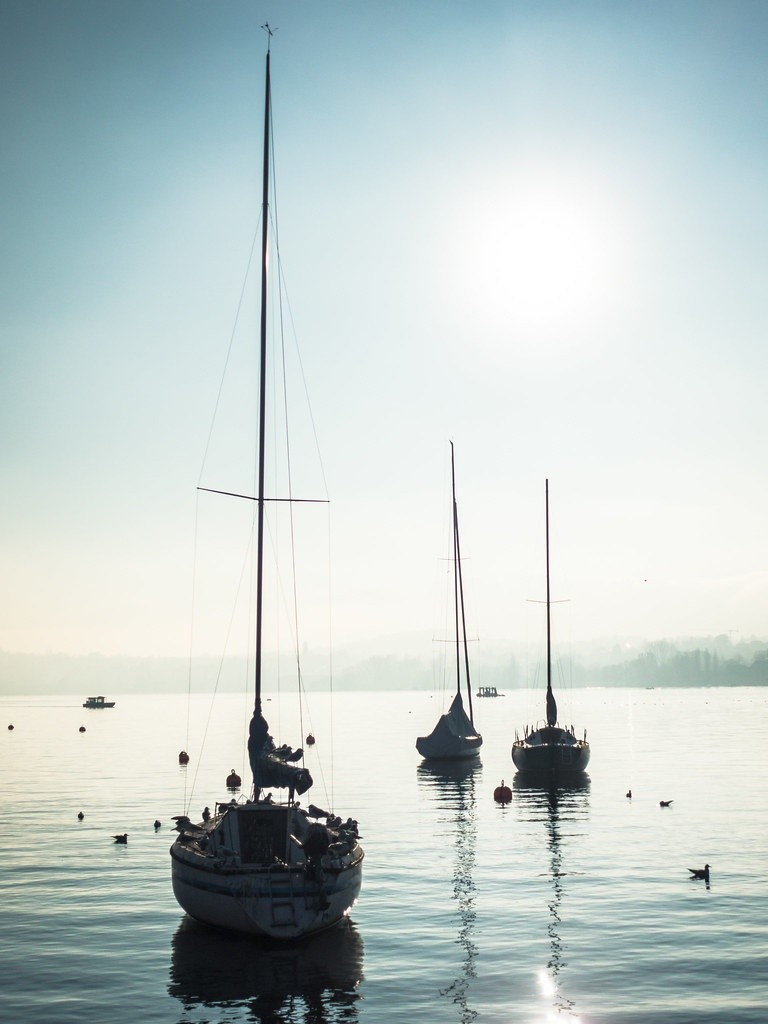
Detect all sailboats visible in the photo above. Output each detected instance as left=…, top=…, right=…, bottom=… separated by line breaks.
left=171, top=23, right=371, bottom=946
left=512, top=481, right=594, bottom=776
left=415, top=436, right=485, bottom=762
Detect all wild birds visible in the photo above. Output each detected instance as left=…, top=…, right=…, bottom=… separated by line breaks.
left=79, top=726, right=86, bottom=733
left=111, top=834, right=129, bottom=844
left=154, top=820, right=161, bottom=830
left=263, top=736, right=305, bottom=763
left=8, top=725, right=14, bottom=730
left=78, top=811, right=85, bottom=821
left=168, top=793, right=363, bottom=871
left=306, top=733, right=316, bottom=748
left=227, top=770, right=241, bottom=787
left=686, top=863, right=712, bottom=880
left=179, top=751, right=189, bottom=765
left=659, top=799, right=675, bottom=808
left=626, top=790, right=632, bottom=799
left=493, top=780, right=512, bottom=807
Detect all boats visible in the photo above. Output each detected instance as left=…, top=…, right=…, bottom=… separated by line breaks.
left=83, top=695, right=116, bottom=709
left=476, top=686, right=504, bottom=698
